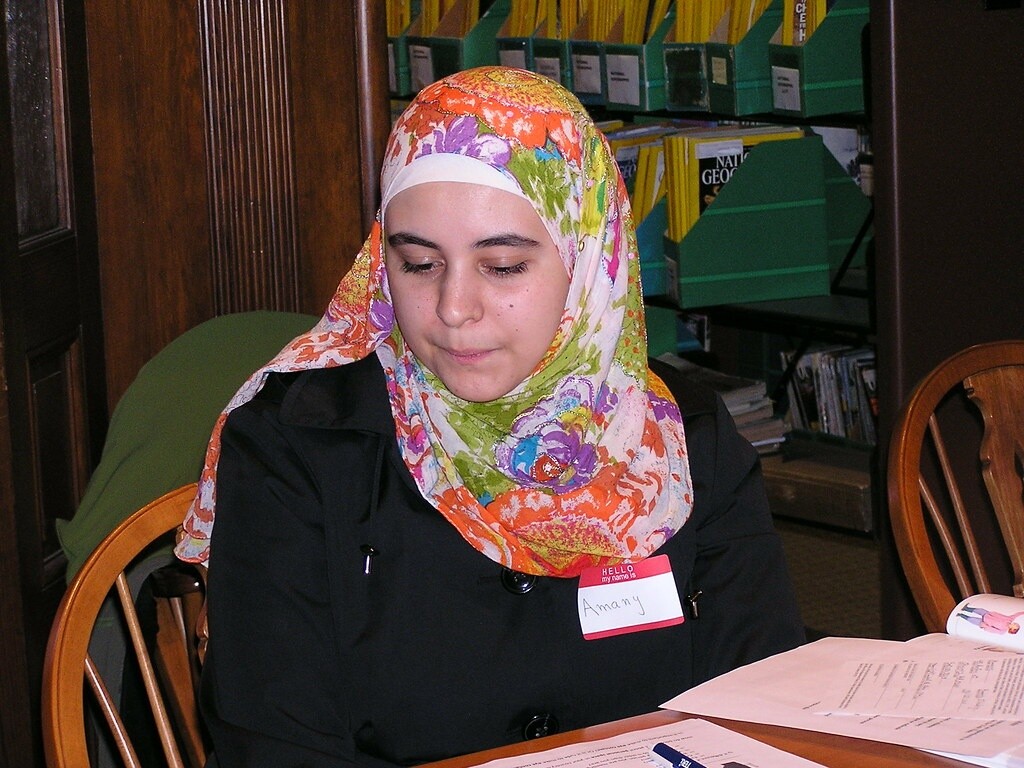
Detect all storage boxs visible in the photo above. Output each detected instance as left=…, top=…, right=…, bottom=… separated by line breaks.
left=431, top=0, right=512, bottom=84
left=664, top=134, right=832, bottom=308
left=533, top=16, right=571, bottom=92
left=387, top=0, right=421, bottom=98
left=496, top=10, right=533, bottom=71
left=766, top=0, right=872, bottom=118
left=569, top=12, right=605, bottom=106
left=707, top=0, right=785, bottom=117
left=757, top=452, right=874, bottom=531
left=604, top=0, right=677, bottom=111
left=807, top=127, right=872, bottom=269
left=662, top=19, right=718, bottom=111
left=635, top=191, right=669, bottom=296
left=406, top=13, right=437, bottom=95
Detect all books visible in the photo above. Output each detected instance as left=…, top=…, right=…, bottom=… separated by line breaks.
left=779, top=341, right=878, bottom=447
left=654, top=352, right=794, bottom=456
left=594, top=120, right=806, bottom=242
left=386, top=0, right=826, bottom=47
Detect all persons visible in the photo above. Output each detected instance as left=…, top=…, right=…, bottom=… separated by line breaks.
left=53, top=309, right=322, bottom=768
left=173, top=65, right=808, bottom=768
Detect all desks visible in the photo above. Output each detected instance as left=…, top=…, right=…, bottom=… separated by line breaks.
left=413, top=710, right=988, bottom=768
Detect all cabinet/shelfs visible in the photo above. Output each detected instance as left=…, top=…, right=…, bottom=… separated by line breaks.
left=352, top=0, right=907, bottom=564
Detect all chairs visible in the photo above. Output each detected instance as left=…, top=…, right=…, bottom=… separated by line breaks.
left=886, top=338, right=1024, bottom=633
left=41, top=483, right=209, bottom=768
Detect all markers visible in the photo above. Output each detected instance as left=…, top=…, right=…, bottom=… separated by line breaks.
left=650, top=742, right=707, bottom=768
